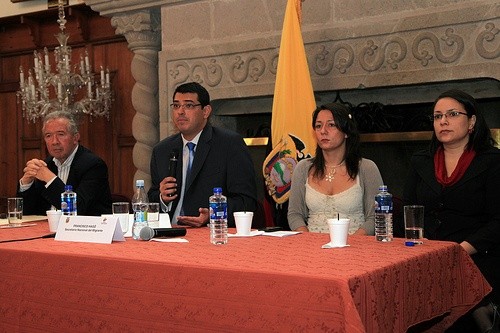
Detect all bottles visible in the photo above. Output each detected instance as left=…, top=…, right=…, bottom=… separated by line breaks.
left=209, top=188, right=228, bottom=246
left=131, top=179, right=150, bottom=226
left=61, top=185, right=78, bottom=216
left=374, top=185, right=393, bottom=243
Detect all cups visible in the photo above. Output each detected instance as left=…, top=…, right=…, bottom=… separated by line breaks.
left=146, top=202, right=159, bottom=228
left=404, top=204, right=424, bottom=245
left=7, top=197, right=23, bottom=227
left=233, top=211, right=254, bottom=236
left=327, top=218, right=350, bottom=246
left=46, top=210, right=61, bottom=233
left=112, top=202, right=129, bottom=235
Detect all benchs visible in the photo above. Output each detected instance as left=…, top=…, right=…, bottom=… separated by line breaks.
left=243, top=130, right=500, bottom=227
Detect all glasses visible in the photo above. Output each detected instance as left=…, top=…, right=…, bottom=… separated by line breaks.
left=168, top=102, right=203, bottom=109
left=434, top=111, right=469, bottom=120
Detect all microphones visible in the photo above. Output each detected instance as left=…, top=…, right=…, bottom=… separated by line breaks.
left=167, top=146, right=179, bottom=200
left=139, top=227, right=187, bottom=240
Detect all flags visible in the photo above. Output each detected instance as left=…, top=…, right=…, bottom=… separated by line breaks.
left=262, top=0, right=319, bottom=231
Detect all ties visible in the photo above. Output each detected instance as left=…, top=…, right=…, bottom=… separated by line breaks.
left=185, top=142, right=196, bottom=170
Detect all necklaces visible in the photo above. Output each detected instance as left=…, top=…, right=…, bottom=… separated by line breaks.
left=324, top=160, right=344, bottom=182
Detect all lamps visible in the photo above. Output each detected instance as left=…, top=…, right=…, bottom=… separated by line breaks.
left=16, top=0, right=115, bottom=125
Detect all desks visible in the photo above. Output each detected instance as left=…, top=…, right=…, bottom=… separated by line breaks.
left=0, top=220, right=492, bottom=333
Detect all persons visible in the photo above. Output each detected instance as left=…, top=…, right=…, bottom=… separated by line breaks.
left=381, top=88, right=500, bottom=333
left=145, top=82, right=267, bottom=230
left=287, top=103, right=387, bottom=236
left=15, top=111, right=114, bottom=218
